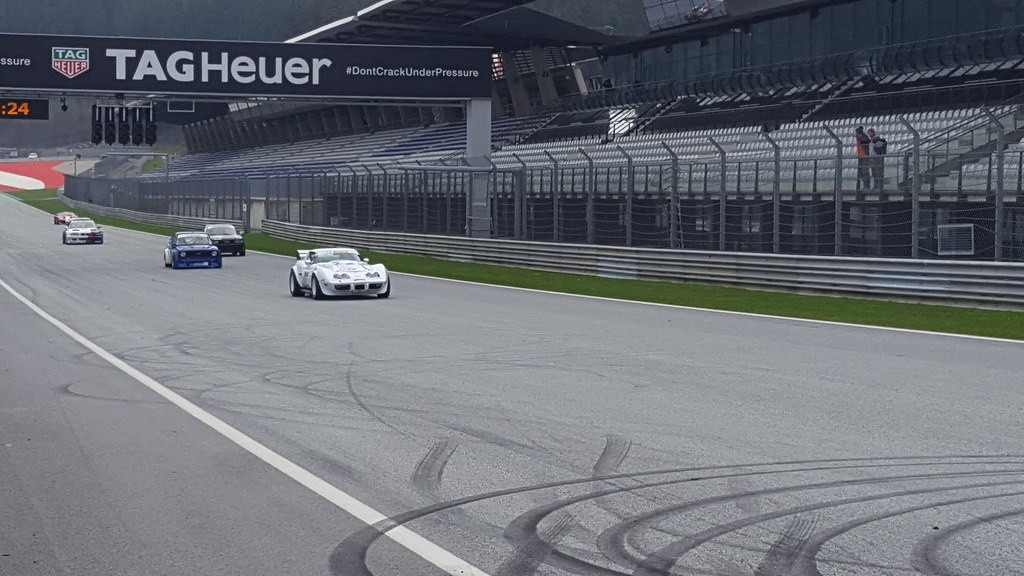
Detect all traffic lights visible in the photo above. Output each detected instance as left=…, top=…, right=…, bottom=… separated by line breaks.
left=118, top=123, right=131, bottom=145
left=105, top=122, right=116, bottom=145
left=134, top=123, right=145, bottom=146
left=146, top=123, right=157, bottom=145
left=92, top=122, right=103, bottom=145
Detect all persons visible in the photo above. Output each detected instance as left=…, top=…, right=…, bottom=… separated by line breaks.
left=867, top=129, right=886, bottom=189
left=602, top=79, right=612, bottom=106
left=855, top=126, right=871, bottom=190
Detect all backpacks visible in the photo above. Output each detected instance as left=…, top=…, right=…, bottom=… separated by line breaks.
left=872, top=137, right=887, bottom=154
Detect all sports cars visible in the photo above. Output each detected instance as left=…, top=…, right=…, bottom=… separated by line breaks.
left=54, top=212, right=79, bottom=224
left=62, top=218, right=103, bottom=245
left=288, top=247, right=391, bottom=299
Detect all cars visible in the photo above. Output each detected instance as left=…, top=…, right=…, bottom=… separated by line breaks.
left=164, top=231, right=222, bottom=268
left=203, top=224, right=246, bottom=256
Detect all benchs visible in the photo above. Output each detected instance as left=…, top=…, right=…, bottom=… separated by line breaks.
left=133, top=114, right=554, bottom=193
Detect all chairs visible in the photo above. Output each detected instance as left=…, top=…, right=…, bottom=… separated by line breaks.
left=490, top=106, right=1024, bottom=198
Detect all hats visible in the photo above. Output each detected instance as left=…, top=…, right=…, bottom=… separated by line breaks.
left=868, top=128, right=875, bottom=133
left=856, top=125, right=864, bottom=133
